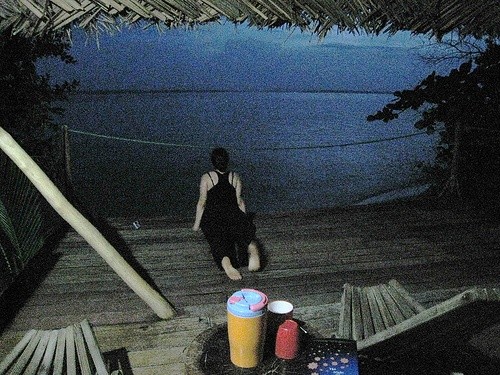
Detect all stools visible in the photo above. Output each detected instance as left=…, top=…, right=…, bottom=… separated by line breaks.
left=0, top=319, right=111, bottom=375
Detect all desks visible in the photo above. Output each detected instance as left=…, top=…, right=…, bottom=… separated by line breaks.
left=180, top=314, right=325, bottom=375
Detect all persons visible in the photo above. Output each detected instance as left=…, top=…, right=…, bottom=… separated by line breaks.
left=192, top=147, right=261, bottom=281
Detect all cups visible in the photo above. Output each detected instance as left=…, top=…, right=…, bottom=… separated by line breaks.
left=274, top=320, right=299, bottom=359
left=267, top=300, right=294, bottom=350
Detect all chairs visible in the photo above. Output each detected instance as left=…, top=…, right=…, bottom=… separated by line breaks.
left=336, top=278, right=500, bottom=375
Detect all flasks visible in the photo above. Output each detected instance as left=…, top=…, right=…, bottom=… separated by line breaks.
left=227, top=289, right=269, bottom=368
